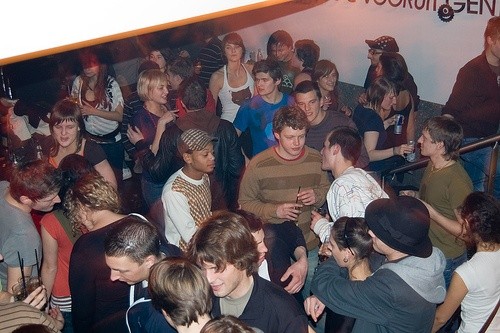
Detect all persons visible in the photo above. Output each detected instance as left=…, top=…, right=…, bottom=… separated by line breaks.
left=64, top=171, right=165, bottom=333
left=235, top=208, right=268, bottom=268
left=432, top=191, right=500, bottom=333
left=162, top=128, right=218, bottom=255
left=36, top=99, right=117, bottom=191
left=40, top=153, right=97, bottom=332
left=171, top=22, right=421, bottom=191
left=0, top=159, right=61, bottom=293
left=70, top=47, right=126, bottom=185
left=127, top=78, right=245, bottom=208
left=1, top=302, right=64, bottom=332
left=150, top=46, right=169, bottom=80
left=103, top=217, right=185, bottom=332
left=309, top=125, right=390, bottom=243
left=187, top=211, right=309, bottom=332
left=304, top=195, right=446, bottom=332
left=199, top=313, right=255, bottom=332
left=133, top=69, right=179, bottom=205
left=441, top=15, right=499, bottom=200
left=164, top=56, right=216, bottom=116
left=238, top=106, right=334, bottom=249
left=148, top=257, right=264, bottom=332
left=123, top=60, right=174, bottom=167
left=325, top=215, right=372, bottom=332
left=399, top=114, right=475, bottom=288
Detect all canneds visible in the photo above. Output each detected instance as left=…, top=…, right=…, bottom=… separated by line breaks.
left=394, top=115, right=403, bottom=134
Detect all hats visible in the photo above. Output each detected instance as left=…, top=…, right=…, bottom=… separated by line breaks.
left=365, top=36, right=399, bottom=52
left=177, top=128, right=218, bottom=155
left=365, top=195, right=433, bottom=258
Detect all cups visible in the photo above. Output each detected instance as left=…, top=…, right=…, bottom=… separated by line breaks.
left=289, top=206, right=300, bottom=226
left=12, top=275, right=47, bottom=312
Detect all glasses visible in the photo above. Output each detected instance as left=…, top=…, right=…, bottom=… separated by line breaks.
left=368, top=49, right=383, bottom=55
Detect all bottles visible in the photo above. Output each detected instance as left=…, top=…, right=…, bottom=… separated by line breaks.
left=257, top=48, right=263, bottom=62
left=250, top=50, right=255, bottom=63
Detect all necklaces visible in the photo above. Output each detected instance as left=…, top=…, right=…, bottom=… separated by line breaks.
left=427, top=159, right=453, bottom=176
